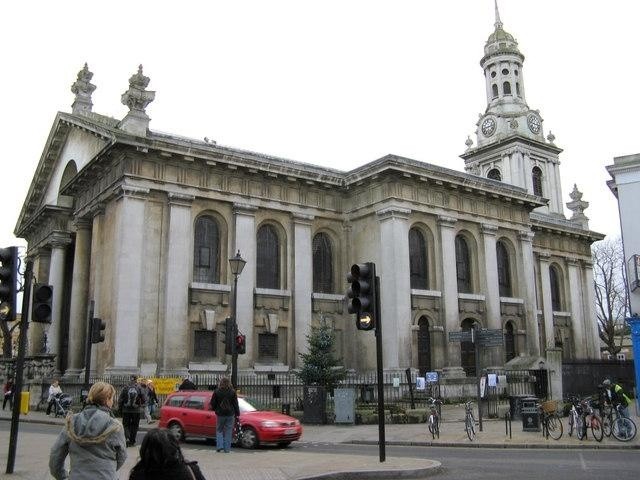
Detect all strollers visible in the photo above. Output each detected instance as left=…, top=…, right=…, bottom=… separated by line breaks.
left=53, top=391, right=73, bottom=419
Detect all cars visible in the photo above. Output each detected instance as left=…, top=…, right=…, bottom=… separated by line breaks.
left=156, top=390, right=303, bottom=447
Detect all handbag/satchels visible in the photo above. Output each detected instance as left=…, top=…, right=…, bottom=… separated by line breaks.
left=233, top=421, right=244, bottom=440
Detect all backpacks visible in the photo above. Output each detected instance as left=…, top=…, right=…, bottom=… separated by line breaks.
left=123, top=385, right=142, bottom=409
left=214, top=391, right=234, bottom=415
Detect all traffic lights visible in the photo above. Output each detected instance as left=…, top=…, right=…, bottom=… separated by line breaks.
left=0, top=246, right=18, bottom=322
left=342, top=260, right=379, bottom=330
left=31, top=282, right=54, bottom=326
left=90, top=317, right=107, bottom=344
left=233, top=334, right=248, bottom=355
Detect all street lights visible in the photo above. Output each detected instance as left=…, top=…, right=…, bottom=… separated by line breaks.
left=227, top=250, right=247, bottom=389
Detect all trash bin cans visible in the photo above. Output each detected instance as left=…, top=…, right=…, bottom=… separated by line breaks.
left=521, top=399, right=541, bottom=432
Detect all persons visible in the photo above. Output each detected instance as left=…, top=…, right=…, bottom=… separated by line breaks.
left=46, top=380, right=63, bottom=417
left=210, top=377, right=240, bottom=453
left=48, top=381, right=128, bottom=480
left=3, top=377, right=14, bottom=410
left=602, top=379, right=634, bottom=438
left=177, top=377, right=198, bottom=392
left=128, top=428, right=205, bottom=480
left=117, top=376, right=159, bottom=448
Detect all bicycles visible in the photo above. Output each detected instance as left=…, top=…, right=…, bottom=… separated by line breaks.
left=564, top=394, right=581, bottom=438
left=579, top=401, right=604, bottom=442
left=603, top=402, right=637, bottom=442
left=541, top=398, right=565, bottom=441
left=457, top=399, right=479, bottom=444
left=424, top=396, right=444, bottom=440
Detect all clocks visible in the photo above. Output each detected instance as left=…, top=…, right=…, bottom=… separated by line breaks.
left=527, top=113, right=541, bottom=134
left=481, top=117, right=496, bottom=137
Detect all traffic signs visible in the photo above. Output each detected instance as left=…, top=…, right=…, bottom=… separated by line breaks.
left=449, top=326, right=505, bottom=349
